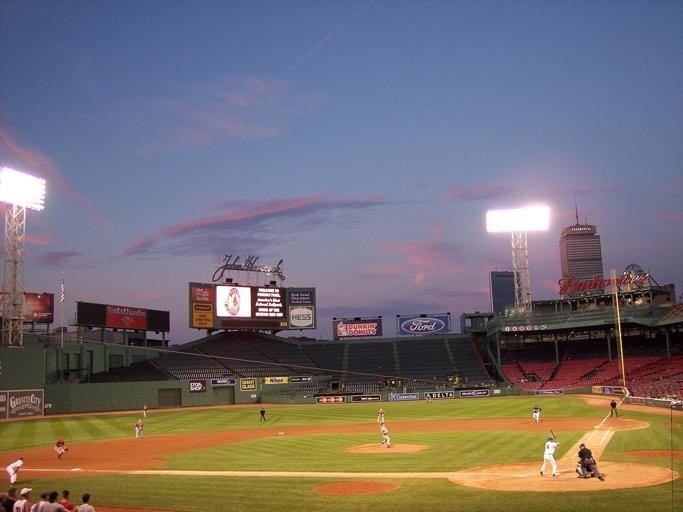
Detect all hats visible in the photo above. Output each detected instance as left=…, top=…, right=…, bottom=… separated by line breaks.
left=8, top=487, right=16, bottom=493
left=20, top=488, right=32, bottom=495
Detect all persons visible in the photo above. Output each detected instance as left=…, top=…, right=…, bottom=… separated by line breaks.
left=578, top=444, right=604, bottom=481
left=375, top=408, right=385, bottom=424
left=135, top=420, right=144, bottom=439
left=379, top=425, right=391, bottom=447
left=142, top=403, right=147, bottom=418
left=540, top=434, right=559, bottom=477
left=532, top=404, right=541, bottom=423
left=259, top=408, right=265, bottom=422
left=55, top=437, right=68, bottom=462
left=7, top=456, right=25, bottom=486
left=609, top=400, right=618, bottom=418
left=575, top=459, right=603, bottom=477
left=0, top=487, right=96, bottom=511
left=425, top=393, right=432, bottom=403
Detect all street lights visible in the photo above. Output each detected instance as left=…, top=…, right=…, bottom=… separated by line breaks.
left=0, top=169, right=47, bottom=351
left=484, top=205, right=551, bottom=319
